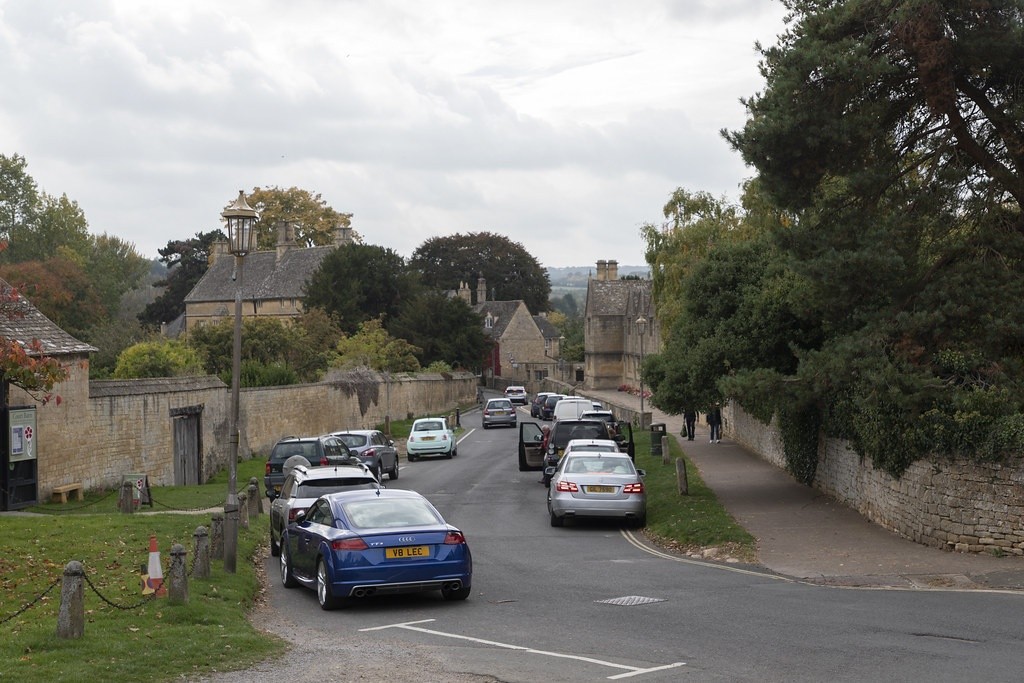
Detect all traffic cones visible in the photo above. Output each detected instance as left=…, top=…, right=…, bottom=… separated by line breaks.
left=139, top=564, right=157, bottom=601
left=148, top=533, right=169, bottom=601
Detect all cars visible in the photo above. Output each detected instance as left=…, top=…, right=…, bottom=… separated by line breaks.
left=548, top=438, right=622, bottom=473
left=530, top=392, right=558, bottom=418
left=545, top=451, right=648, bottom=529
left=479, top=398, right=517, bottom=429
left=406, top=417, right=458, bottom=461
left=537, top=393, right=567, bottom=421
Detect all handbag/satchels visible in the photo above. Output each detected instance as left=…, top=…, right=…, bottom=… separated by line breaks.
left=719, top=427, right=723, bottom=439
left=680, top=422, right=687, bottom=437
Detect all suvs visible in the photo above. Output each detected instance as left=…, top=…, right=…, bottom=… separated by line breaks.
left=264, top=435, right=358, bottom=503
left=503, top=385, right=530, bottom=405
left=270, top=462, right=386, bottom=557
left=518, top=417, right=635, bottom=488
left=323, top=430, right=399, bottom=485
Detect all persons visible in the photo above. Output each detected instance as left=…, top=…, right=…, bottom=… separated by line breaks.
left=707, top=402, right=722, bottom=444
left=683, top=410, right=699, bottom=441
left=537, top=424, right=552, bottom=483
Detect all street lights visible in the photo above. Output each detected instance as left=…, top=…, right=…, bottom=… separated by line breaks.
left=635, top=314, right=649, bottom=430
left=511, top=358, right=514, bottom=386
left=559, top=334, right=567, bottom=381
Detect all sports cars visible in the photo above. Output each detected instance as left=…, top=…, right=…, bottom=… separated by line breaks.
left=278, top=489, right=473, bottom=611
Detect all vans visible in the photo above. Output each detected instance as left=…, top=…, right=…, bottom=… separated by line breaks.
left=548, top=395, right=624, bottom=438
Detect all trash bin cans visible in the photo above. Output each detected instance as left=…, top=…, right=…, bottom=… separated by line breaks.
left=650, top=423, right=667, bottom=456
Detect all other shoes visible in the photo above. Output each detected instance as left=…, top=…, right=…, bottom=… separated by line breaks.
left=710, top=440, right=713, bottom=444
left=538, top=477, right=545, bottom=483
left=716, top=440, right=720, bottom=443
